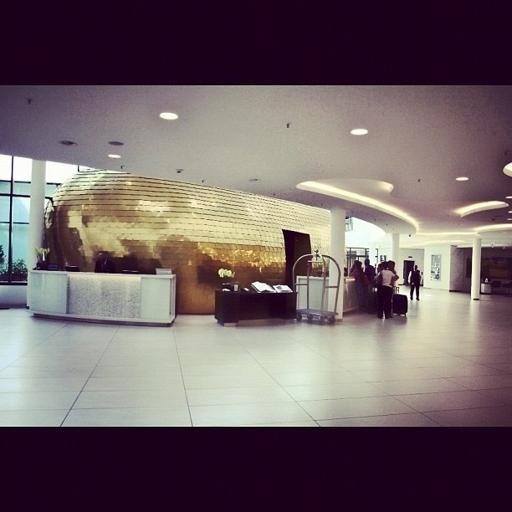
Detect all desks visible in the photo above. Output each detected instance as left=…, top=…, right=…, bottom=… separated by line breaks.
left=27, top=265, right=176, bottom=325
left=214, top=289, right=297, bottom=327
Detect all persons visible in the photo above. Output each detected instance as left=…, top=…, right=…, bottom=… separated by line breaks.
left=351, top=258, right=399, bottom=319
left=408, top=265, right=420, bottom=300
left=94, top=251, right=115, bottom=273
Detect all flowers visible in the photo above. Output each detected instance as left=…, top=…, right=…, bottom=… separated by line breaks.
left=218, top=268, right=235, bottom=279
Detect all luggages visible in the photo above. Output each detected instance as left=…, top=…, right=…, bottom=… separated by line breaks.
left=393, top=287, right=407, bottom=314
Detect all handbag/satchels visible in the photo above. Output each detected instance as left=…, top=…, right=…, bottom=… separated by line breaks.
left=360, top=272, right=369, bottom=286
left=375, top=275, right=382, bottom=288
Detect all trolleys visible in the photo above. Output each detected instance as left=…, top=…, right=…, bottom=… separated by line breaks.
left=292, top=253, right=341, bottom=324
